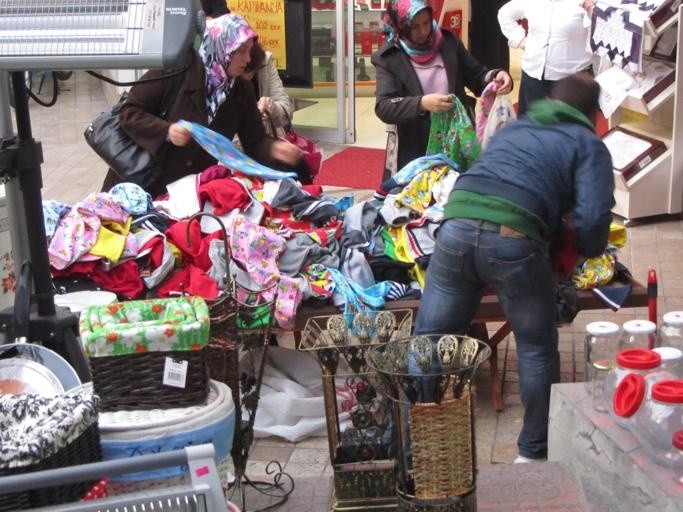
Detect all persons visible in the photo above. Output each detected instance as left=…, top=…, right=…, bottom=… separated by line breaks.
left=397, top=75, right=615, bottom=482
left=99, top=13, right=314, bottom=191
left=496, top=0, right=597, bottom=118
left=370, top=0, right=513, bottom=190
left=190, top=1, right=294, bottom=158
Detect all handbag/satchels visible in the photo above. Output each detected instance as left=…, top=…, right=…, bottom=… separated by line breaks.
left=82, top=56, right=197, bottom=192
left=271, top=101, right=323, bottom=176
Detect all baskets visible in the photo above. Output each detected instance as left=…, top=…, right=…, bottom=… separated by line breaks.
left=0, top=392, right=103, bottom=510
left=80, top=293, right=211, bottom=410
